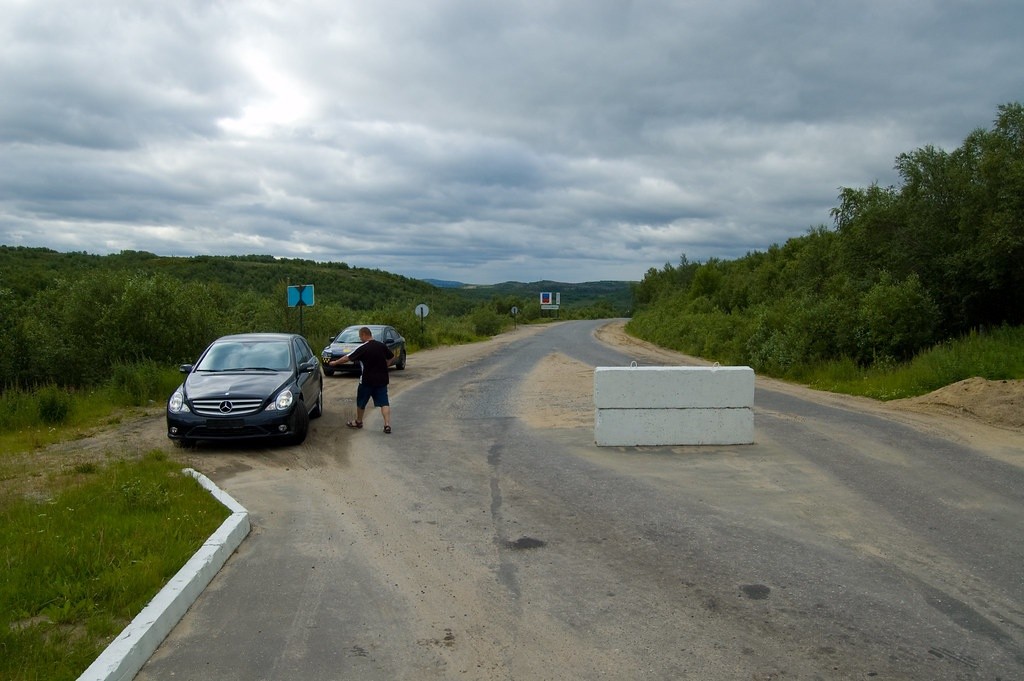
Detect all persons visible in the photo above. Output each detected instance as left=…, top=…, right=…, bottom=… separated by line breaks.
left=329, top=328, right=394, bottom=435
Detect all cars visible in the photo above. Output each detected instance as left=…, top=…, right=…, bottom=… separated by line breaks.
left=321, top=325, right=407, bottom=377
left=165, top=332, right=324, bottom=446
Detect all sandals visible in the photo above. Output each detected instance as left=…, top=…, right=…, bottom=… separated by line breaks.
left=383, top=425, right=391, bottom=433
left=347, top=419, right=363, bottom=429
